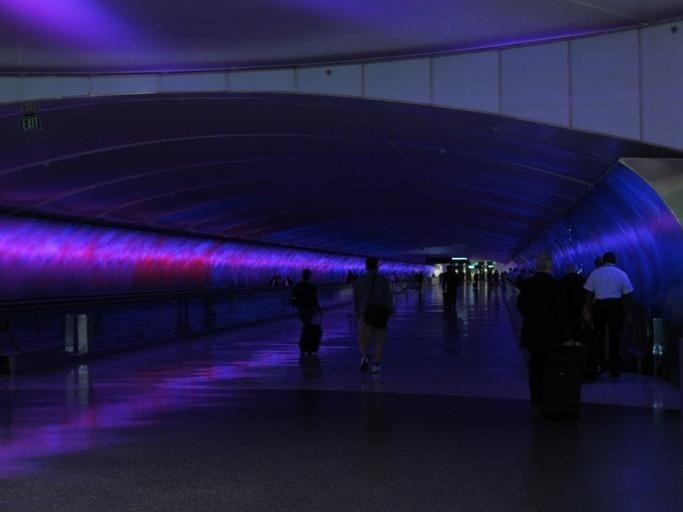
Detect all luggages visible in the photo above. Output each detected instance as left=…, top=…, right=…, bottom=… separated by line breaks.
left=540, top=345, right=582, bottom=422
left=298, top=313, right=322, bottom=355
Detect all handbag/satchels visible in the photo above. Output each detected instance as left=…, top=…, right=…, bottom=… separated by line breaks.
left=363, top=304, right=390, bottom=327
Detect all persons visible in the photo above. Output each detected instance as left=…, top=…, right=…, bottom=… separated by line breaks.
left=344, top=256, right=397, bottom=377
left=267, top=269, right=325, bottom=368
left=415, top=252, right=636, bottom=422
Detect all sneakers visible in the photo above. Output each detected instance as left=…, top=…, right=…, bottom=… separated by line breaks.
left=359, top=353, right=370, bottom=373
left=372, top=364, right=382, bottom=373
